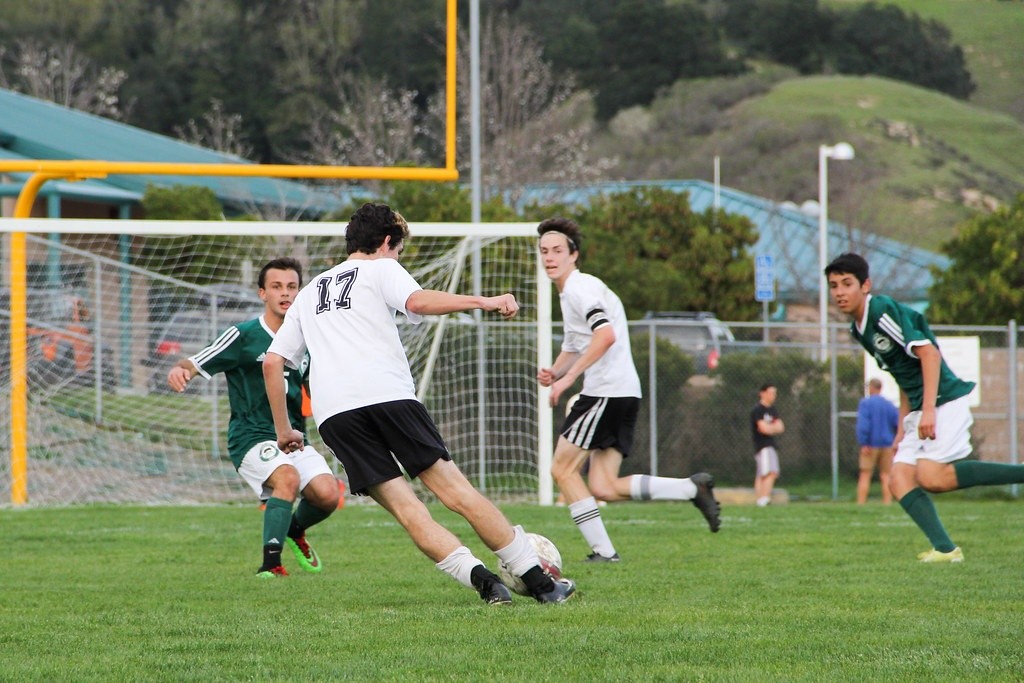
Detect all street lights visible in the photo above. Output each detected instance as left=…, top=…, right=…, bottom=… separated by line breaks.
left=817, top=142, right=857, bottom=495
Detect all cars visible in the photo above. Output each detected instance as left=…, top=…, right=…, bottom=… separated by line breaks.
left=150, top=310, right=261, bottom=397
left=0, top=289, right=118, bottom=403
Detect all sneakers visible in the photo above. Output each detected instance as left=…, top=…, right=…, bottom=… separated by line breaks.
left=286, top=534, right=322, bottom=574
left=582, top=552, right=619, bottom=562
left=538, top=576, right=576, bottom=607
left=917, top=545, right=964, bottom=563
left=468, top=565, right=512, bottom=606
left=257, top=566, right=287, bottom=581
left=690, top=472, right=720, bottom=533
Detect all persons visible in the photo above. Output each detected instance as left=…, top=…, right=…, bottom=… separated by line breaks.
left=749, top=383, right=785, bottom=507
left=168, top=202, right=720, bottom=607
left=824, top=252, right=1024, bottom=565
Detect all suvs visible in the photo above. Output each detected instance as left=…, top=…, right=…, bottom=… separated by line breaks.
left=634, top=310, right=739, bottom=403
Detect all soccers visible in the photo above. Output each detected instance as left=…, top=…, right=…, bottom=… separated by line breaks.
left=502, top=532, right=564, bottom=597
